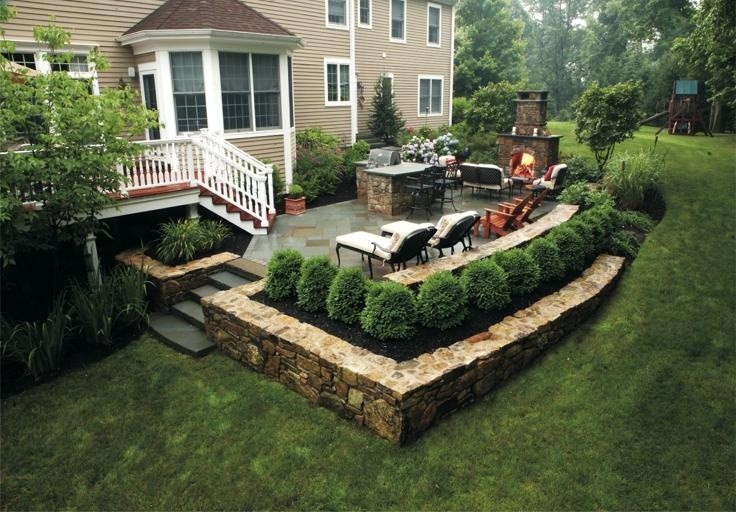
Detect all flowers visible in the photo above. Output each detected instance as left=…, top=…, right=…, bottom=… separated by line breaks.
left=400, top=131, right=460, bottom=166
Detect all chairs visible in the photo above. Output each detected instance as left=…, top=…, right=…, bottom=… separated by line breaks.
left=335, top=162, right=568, bottom=282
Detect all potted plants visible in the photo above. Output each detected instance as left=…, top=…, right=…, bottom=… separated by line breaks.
left=284, top=184, right=306, bottom=215
